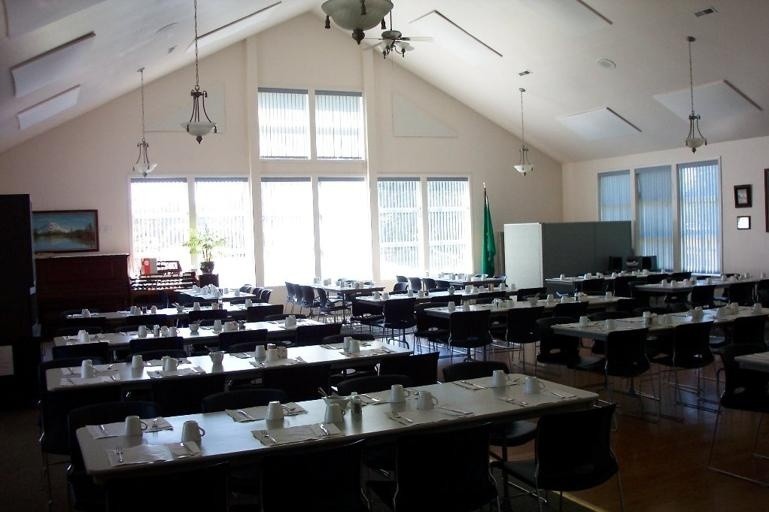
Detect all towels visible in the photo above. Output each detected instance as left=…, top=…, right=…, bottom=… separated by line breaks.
left=339, top=346, right=391, bottom=358
left=453, top=376, right=519, bottom=390
left=251, top=422, right=345, bottom=446
left=60, top=373, right=120, bottom=386
left=321, top=340, right=371, bottom=350
left=147, top=363, right=206, bottom=379
left=250, top=356, right=306, bottom=368
left=225, top=402, right=307, bottom=422
left=60, top=364, right=114, bottom=375
left=63, top=333, right=109, bottom=345
left=385, top=404, right=474, bottom=426
left=230, top=348, right=267, bottom=359
left=86, top=417, right=173, bottom=440
left=107, top=441, right=202, bottom=467
left=499, top=388, right=576, bottom=407
left=361, top=387, right=420, bottom=404
left=149, top=357, right=192, bottom=365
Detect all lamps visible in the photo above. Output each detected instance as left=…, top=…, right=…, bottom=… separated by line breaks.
left=322, top=0, right=394, bottom=44
left=685, top=36, right=707, bottom=154
left=132, top=68, right=158, bottom=177
left=380, top=40, right=409, bottom=59
left=514, top=88, right=535, bottom=177
left=181, top=0, right=217, bottom=143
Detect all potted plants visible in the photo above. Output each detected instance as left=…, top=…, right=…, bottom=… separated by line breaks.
left=183, top=224, right=226, bottom=273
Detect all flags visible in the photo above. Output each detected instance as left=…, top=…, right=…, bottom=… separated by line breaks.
left=480, top=181, right=497, bottom=276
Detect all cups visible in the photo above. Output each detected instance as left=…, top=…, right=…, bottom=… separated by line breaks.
left=418, top=391, right=439, bottom=410
left=80, top=360, right=98, bottom=380
left=327, top=405, right=347, bottom=422
left=390, top=385, right=411, bottom=403
left=193, top=284, right=241, bottom=297
left=351, top=339, right=361, bottom=354
left=493, top=295, right=518, bottom=309
left=716, top=271, right=769, bottom=283
left=417, top=290, right=426, bottom=298
left=78, top=330, right=91, bottom=344
left=245, top=299, right=253, bottom=310
left=604, top=292, right=613, bottom=302
left=138, top=326, right=148, bottom=338
left=82, top=309, right=91, bottom=318
left=426, top=269, right=489, bottom=282
left=312, top=275, right=374, bottom=289
left=130, top=306, right=141, bottom=315
left=125, top=416, right=148, bottom=437
left=172, top=327, right=178, bottom=338
left=463, top=305, right=470, bottom=312
left=547, top=295, right=556, bottom=305
left=752, top=303, right=762, bottom=315
left=524, top=376, right=547, bottom=395
left=285, top=315, right=298, bottom=328
left=557, top=269, right=652, bottom=279
left=164, top=358, right=180, bottom=373
left=343, top=336, right=353, bottom=354
left=266, top=402, right=292, bottom=421
left=660, top=275, right=713, bottom=288
left=212, top=303, right=219, bottom=310
left=606, top=320, right=618, bottom=330
left=662, top=315, right=674, bottom=325
left=152, top=306, right=158, bottom=314
left=408, top=289, right=415, bottom=298
left=225, top=321, right=237, bottom=332
left=448, top=302, right=456, bottom=313
left=181, top=420, right=206, bottom=443
left=578, top=315, right=592, bottom=329
left=372, top=290, right=390, bottom=300
left=463, top=282, right=520, bottom=293
left=494, top=370, right=511, bottom=387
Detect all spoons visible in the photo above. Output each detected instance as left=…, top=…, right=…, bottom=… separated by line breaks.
left=391, top=410, right=413, bottom=425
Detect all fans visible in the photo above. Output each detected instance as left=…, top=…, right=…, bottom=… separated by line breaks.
left=362, top=30, right=432, bottom=53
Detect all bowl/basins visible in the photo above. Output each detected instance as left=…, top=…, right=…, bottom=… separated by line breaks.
left=527, top=297, right=540, bottom=306
left=322, top=396, right=352, bottom=411
left=176, top=307, right=185, bottom=313
left=208, top=351, right=226, bottom=366
left=189, top=324, right=201, bottom=332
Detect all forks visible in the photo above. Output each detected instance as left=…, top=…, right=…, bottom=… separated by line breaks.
left=261, top=429, right=278, bottom=446
left=361, top=394, right=381, bottom=403
left=385, top=412, right=408, bottom=428
left=462, top=381, right=488, bottom=391
left=116, top=445, right=124, bottom=464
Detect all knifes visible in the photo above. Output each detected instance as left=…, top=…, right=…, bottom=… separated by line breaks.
left=99, top=423, right=112, bottom=439
left=320, top=424, right=334, bottom=435
left=441, top=407, right=467, bottom=416
left=237, top=410, right=255, bottom=422
left=179, top=441, right=195, bottom=458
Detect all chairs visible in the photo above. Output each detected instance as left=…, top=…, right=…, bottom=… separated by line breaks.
left=205, top=389, right=285, bottom=414
left=491, top=401, right=625, bottom=511
left=518, top=287, right=547, bottom=301
left=707, top=340, right=769, bottom=483
left=231, top=284, right=251, bottom=302
left=317, top=288, right=346, bottom=322
left=574, top=327, right=660, bottom=431
left=689, top=285, right=717, bottom=309
left=443, top=360, right=538, bottom=465
left=477, top=291, right=506, bottom=303
left=652, top=320, right=720, bottom=422
left=552, top=301, right=589, bottom=316
left=68, top=317, right=106, bottom=335
left=219, top=329, right=268, bottom=351
left=245, top=304, right=283, bottom=322
left=449, top=310, right=492, bottom=362
left=727, top=282, right=753, bottom=306
left=295, top=324, right=342, bottom=345
left=52, top=342, right=108, bottom=367
left=423, top=278, right=436, bottom=291
left=435, top=281, right=450, bottom=292
left=397, top=276, right=408, bottom=282
left=252, top=287, right=271, bottom=303
left=491, top=306, right=544, bottom=371
left=362, top=421, right=500, bottom=510
left=65, top=400, right=162, bottom=504
left=414, top=303, right=448, bottom=352
left=130, top=337, right=184, bottom=360
left=122, top=314, right=169, bottom=331
left=189, top=310, right=227, bottom=320
left=409, top=277, right=421, bottom=290
left=322, top=334, right=374, bottom=343
left=369, top=297, right=415, bottom=342
left=431, top=295, right=462, bottom=306
left=537, top=316, right=576, bottom=366
left=374, top=351, right=442, bottom=385
left=337, top=375, right=409, bottom=396
left=284, top=281, right=320, bottom=319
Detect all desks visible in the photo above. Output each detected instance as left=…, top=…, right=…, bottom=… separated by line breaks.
left=323, top=285, right=385, bottom=294
left=436, top=276, right=503, bottom=287
left=551, top=304, right=769, bottom=338
left=45, top=340, right=414, bottom=391
left=67, top=302, right=273, bottom=324
left=545, top=270, right=691, bottom=292
left=76, top=373, right=600, bottom=475
left=55, top=317, right=325, bottom=351
left=635, top=277, right=769, bottom=293
left=423, top=295, right=633, bottom=318
left=356, top=287, right=517, bottom=302
left=174, top=289, right=255, bottom=301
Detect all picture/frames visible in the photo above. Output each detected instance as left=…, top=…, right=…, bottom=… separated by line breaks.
left=736, top=216, right=751, bottom=230
left=31, top=209, right=100, bottom=254
left=734, top=185, right=753, bottom=208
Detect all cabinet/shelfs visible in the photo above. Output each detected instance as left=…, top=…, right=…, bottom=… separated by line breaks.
left=504, top=220, right=632, bottom=289
